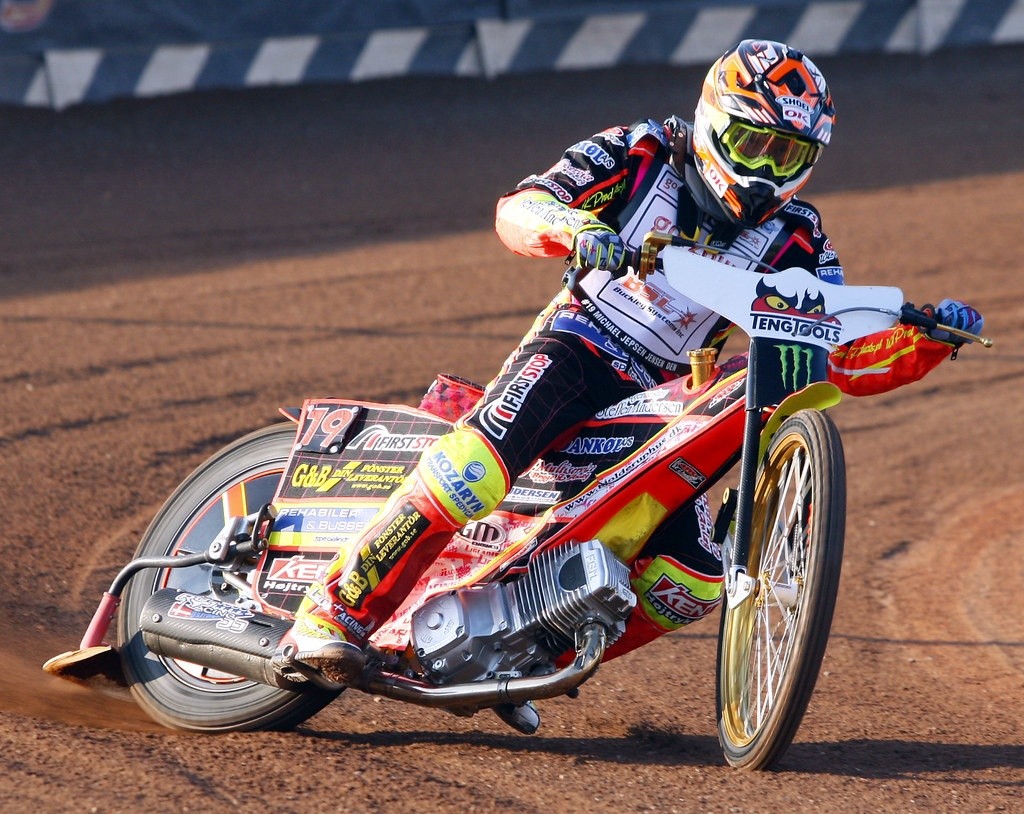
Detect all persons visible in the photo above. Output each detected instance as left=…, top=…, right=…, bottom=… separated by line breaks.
left=269, top=39, right=983, bottom=735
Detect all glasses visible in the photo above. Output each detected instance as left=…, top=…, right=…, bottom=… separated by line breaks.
left=722, top=121, right=810, bottom=183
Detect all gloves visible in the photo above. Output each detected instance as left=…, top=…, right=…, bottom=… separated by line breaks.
left=929, top=298, right=983, bottom=345
left=574, top=228, right=627, bottom=272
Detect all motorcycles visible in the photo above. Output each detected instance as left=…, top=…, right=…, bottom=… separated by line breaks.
left=44, top=231, right=993, bottom=775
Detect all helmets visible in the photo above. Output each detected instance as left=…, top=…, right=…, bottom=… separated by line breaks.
left=692, top=38, right=836, bottom=228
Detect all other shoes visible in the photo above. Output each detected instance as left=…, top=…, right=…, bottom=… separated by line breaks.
left=268, top=617, right=365, bottom=684
left=491, top=700, right=541, bottom=735
